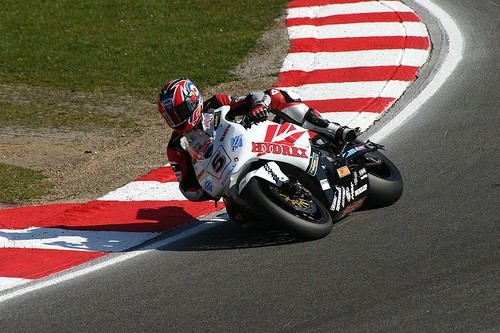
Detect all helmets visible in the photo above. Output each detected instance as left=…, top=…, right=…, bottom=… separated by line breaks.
left=157, top=78, right=203, bottom=134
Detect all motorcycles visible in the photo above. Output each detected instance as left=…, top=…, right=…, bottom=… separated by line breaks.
left=179, top=104, right=404, bottom=241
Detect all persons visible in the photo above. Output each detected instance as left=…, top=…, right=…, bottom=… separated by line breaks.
left=157, top=77, right=357, bottom=226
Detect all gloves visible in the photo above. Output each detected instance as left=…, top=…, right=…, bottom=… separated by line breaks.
left=248, top=101, right=268, bottom=123
left=203, top=188, right=221, bottom=202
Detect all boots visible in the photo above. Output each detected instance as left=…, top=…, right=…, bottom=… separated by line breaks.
left=301, top=106, right=361, bottom=143
